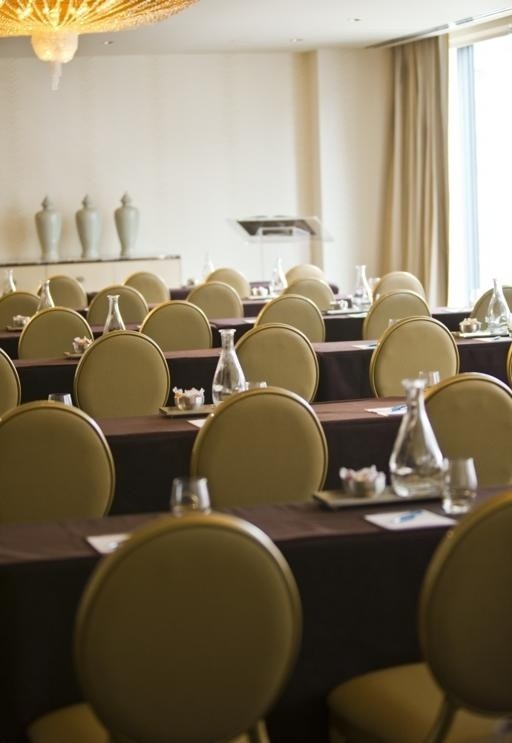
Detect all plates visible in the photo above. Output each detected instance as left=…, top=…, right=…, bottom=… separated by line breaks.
left=7, top=325, right=23, bottom=332
left=62, top=350, right=84, bottom=361
left=326, top=308, right=369, bottom=315
left=247, top=296, right=281, bottom=302
left=458, top=330, right=511, bottom=339
left=160, top=403, right=215, bottom=417
left=312, top=486, right=461, bottom=511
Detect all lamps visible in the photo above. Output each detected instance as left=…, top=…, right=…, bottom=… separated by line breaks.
left=234, top=213, right=333, bottom=298
left=0, top=0, right=199, bottom=92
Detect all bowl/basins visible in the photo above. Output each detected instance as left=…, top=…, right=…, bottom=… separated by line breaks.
left=344, top=473, right=386, bottom=497
left=174, top=394, right=204, bottom=411
left=460, top=320, right=482, bottom=333
left=71, top=342, right=91, bottom=352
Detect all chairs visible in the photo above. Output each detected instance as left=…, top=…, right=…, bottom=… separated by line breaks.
left=1, top=349, right=22, bottom=418
left=253, top=294, right=326, bottom=344
left=1, top=291, right=44, bottom=331
left=18, top=306, right=94, bottom=360
left=362, top=290, right=430, bottom=339
left=0, top=401, right=116, bottom=524
left=420, top=370, right=512, bottom=489
left=372, top=270, right=425, bottom=305
left=283, top=278, right=335, bottom=314
left=86, top=285, right=148, bottom=327
left=471, top=287, right=512, bottom=329
left=188, top=385, right=329, bottom=509
left=139, top=300, right=213, bottom=350
left=72, top=330, right=171, bottom=418
left=285, top=264, right=321, bottom=284
left=207, top=267, right=250, bottom=299
left=124, top=272, right=171, bottom=303
left=369, top=315, right=460, bottom=398
left=37, top=276, right=87, bottom=311
left=233, top=322, right=320, bottom=405
left=327, top=492, right=512, bottom=742
left=184, top=281, right=244, bottom=319
left=22, top=511, right=305, bottom=743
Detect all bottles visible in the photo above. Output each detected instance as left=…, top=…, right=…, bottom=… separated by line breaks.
left=102, top=294, right=127, bottom=336
left=2, top=269, right=17, bottom=297
left=202, top=254, right=215, bottom=281
left=351, top=265, right=373, bottom=311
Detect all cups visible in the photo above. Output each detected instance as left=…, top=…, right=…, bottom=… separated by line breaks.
left=170, top=476, right=211, bottom=521
left=50, top=393, right=75, bottom=408
left=416, top=371, right=441, bottom=390
left=442, top=455, right=476, bottom=517
left=245, top=380, right=267, bottom=392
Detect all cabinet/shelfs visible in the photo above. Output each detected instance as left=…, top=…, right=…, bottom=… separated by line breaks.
left=1, top=254, right=181, bottom=267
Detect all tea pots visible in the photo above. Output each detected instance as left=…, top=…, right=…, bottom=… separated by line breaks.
left=103, top=295, right=127, bottom=332
left=4, top=269, right=17, bottom=295
left=355, top=266, right=372, bottom=310
